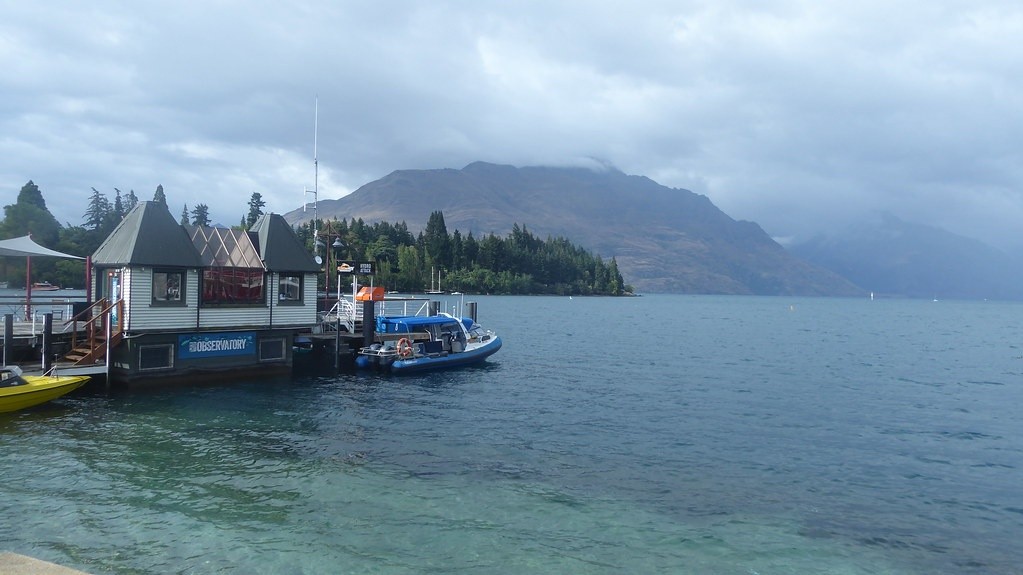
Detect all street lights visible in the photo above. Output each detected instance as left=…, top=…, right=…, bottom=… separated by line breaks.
left=313, top=220, right=345, bottom=311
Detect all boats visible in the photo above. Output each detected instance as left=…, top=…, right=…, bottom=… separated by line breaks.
left=451, top=291, right=464, bottom=295
left=426, top=267, right=445, bottom=294
left=0, top=365, right=94, bottom=413
left=355, top=312, right=503, bottom=376
left=387, top=290, right=399, bottom=295
left=22, top=280, right=60, bottom=291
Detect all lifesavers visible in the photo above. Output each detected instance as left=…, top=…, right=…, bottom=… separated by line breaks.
left=397, top=338, right=411, bottom=356
